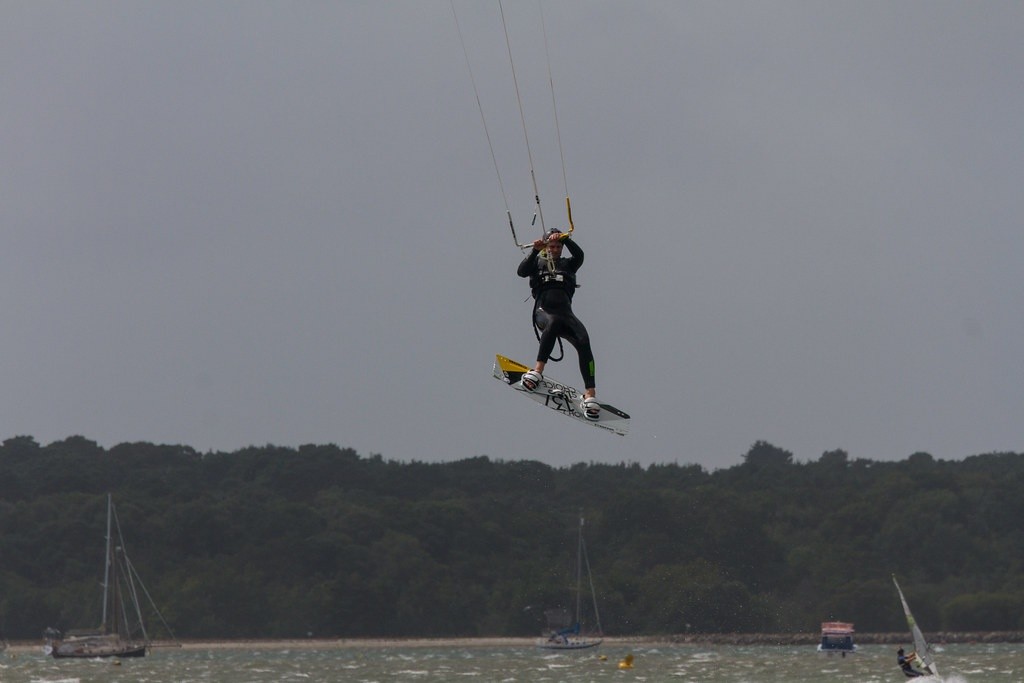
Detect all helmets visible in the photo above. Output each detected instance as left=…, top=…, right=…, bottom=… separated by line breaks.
left=541, top=227, right=562, bottom=242
left=897, top=648, right=904, bottom=653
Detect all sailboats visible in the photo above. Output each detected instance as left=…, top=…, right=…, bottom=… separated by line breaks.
left=892, top=576, right=964, bottom=683
left=44, top=493, right=181, bottom=658
left=537, top=508, right=604, bottom=650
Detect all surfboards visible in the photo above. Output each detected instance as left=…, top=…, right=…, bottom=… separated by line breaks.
left=491, top=354, right=631, bottom=438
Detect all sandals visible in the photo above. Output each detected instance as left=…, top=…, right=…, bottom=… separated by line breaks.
left=520, top=368, right=543, bottom=394
left=580, top=394, right=601, bottom=421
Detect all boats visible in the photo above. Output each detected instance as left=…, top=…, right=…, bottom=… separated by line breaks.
left=817, top=619, right=861, bottom=658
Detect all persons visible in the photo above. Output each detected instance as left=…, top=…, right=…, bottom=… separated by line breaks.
left=516, top=227, right=600, bottom=421
left=896, top=649, right=924, bottom=677
